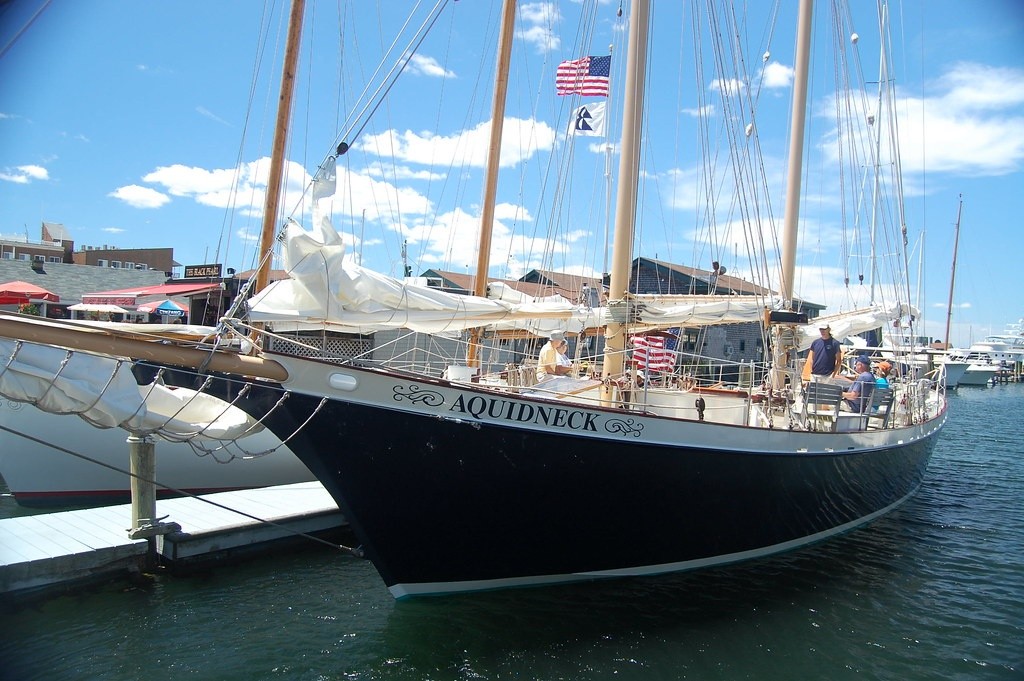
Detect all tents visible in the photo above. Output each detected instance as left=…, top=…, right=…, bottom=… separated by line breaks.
left=0, top=279, right=61, bottom=319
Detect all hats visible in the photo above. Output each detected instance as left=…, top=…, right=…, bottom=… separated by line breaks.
left=548, top=330, right=565, bottom=341
left=872, top=362, right=893, bottom=374
left=853, top=355, right=871, bottom=365
left=818, top=323, right=829, bottom=329
left=636, top=370, right=645, bottom=379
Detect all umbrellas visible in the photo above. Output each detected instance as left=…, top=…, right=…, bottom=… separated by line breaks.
left=136, top=300, right=189, bottom=324
left=67, top=303, right=128, bottom=320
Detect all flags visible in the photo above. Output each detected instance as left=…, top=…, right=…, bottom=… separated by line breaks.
left=567, top=101, right=608, bottom=138
left=633, top=329, right=681, bottom=372
left=554, top=54, right=611, bottom=97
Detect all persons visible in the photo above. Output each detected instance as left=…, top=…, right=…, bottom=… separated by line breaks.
left=537, top=329, right=564, bottom=381
left=809, top=323, right=843, bottom=383
left=240, top=321, right=266, bottom=355
left=555, top=340, right=573, bottom=375
left=868, top=360, right=892, bottom=414
left=822, top=354, right=875, bottom=411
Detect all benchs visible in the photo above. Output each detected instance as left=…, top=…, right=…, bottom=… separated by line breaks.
left=790, top=382, right=843, bottom=432
left=863, top=388, right=894, bottom=430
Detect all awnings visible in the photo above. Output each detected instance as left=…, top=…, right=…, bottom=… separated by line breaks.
left=81, top=283, right=224, bottom=305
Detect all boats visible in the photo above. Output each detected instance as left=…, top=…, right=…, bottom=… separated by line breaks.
left=0, top=0, right=1024, bottom=611
